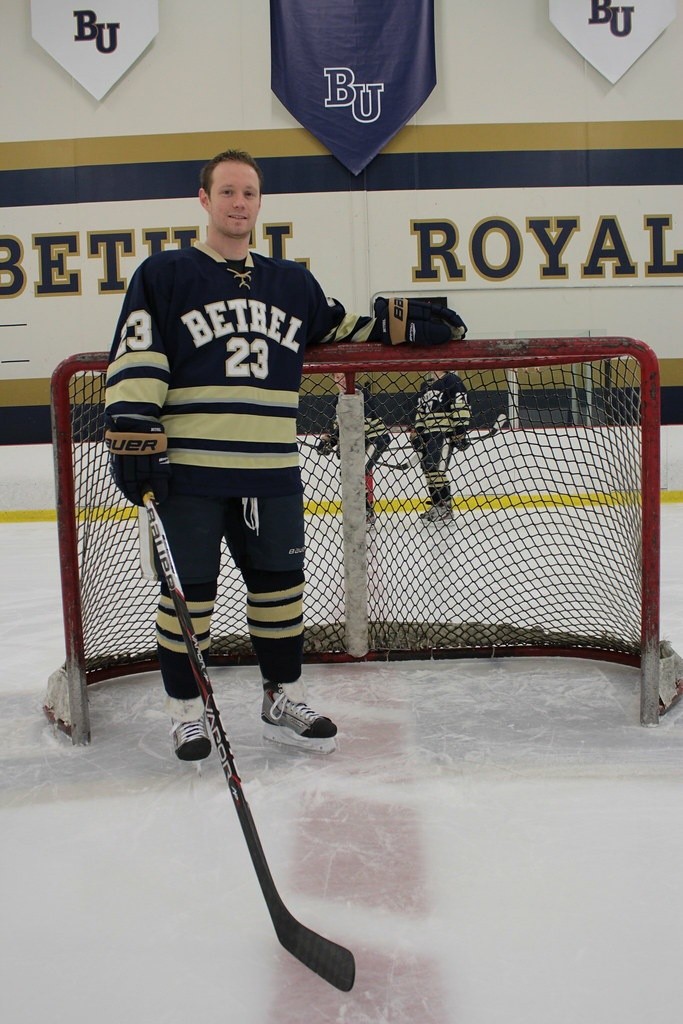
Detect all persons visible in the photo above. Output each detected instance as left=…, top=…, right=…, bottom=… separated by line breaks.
left=102, top=150, right=468, bottom=771
left=316, top=373, right=390, bottom=530
left=410, top=370, right=473, bottom=523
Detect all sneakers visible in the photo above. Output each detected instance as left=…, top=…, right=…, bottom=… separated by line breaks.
left=418, top=498, right=455, bottom=527
left=366, top=512, right=377, bottom=532
left=171, top=714, right=211, bottom=775
left=260, top=676, right=338, bottom=753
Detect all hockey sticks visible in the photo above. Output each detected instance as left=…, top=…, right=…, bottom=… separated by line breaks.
left=145, top=496, right=356, bottom=993
left=295, top=414, right=507, bottom=470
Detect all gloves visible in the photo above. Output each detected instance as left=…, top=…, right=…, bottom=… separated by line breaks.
left=455, top=435, right=471, bottom=451
left=317, top=436, right=332, bottom=456
left=412, top=434, right=429, bottom=453
left=102, top=414, right=171, bottom=509
left=374, top=293, right=469, bottom=346
